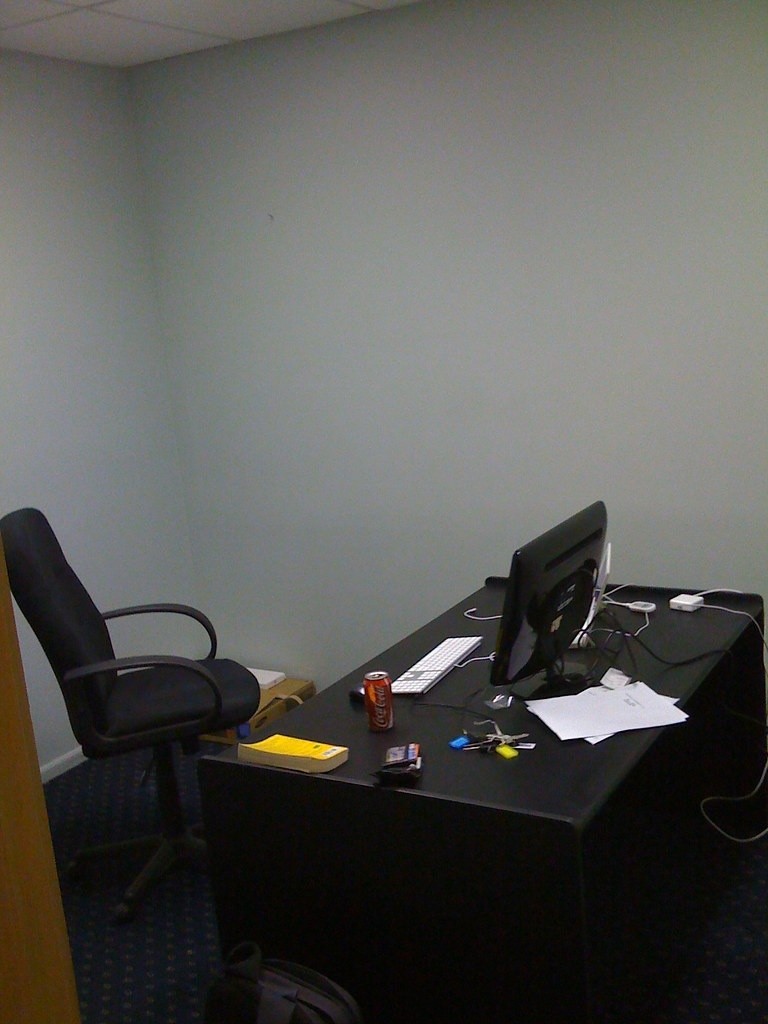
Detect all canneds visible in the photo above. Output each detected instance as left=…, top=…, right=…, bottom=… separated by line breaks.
left=363, top=671, right=394, bottom=732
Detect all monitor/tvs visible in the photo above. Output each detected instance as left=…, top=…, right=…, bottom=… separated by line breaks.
left=487, top=502, right=608, bottom=701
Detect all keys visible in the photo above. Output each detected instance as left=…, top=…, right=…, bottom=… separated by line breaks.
left=463, top=733, right=529, bottom=750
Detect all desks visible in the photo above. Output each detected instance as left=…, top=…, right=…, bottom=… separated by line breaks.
left=198, top=575, right=768, bottom=1024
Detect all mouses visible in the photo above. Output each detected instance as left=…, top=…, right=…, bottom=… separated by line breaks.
left=348, top=687, right=365, bottom=703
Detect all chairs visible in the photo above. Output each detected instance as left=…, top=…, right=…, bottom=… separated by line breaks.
left=0, top=507, right=261, bottom=926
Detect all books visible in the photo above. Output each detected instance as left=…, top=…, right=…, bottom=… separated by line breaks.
left=238, top=734, right=349, bottom=772
left=247, top=668, right=286, bottom=689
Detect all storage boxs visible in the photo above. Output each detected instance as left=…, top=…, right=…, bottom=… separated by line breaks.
left=200, top=676, right=317, bottom=750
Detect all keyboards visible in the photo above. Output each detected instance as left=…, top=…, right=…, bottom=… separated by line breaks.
left=390, top=636, right=484, bottom=695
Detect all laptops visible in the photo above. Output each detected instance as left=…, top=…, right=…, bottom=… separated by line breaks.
left=569, top=543, right=612, bottom=649
